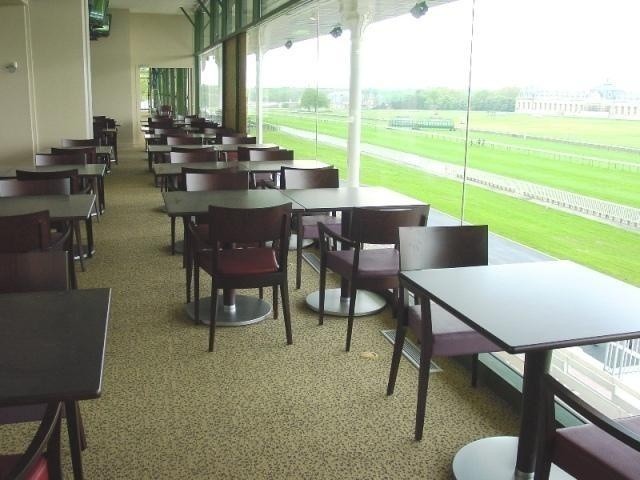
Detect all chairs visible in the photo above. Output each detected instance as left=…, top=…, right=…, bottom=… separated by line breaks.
left=0, top=116, right=105, bottom=480
left=186, top=172, right=264, bottom=303
left=284, top=167, right=343, bottom=288
left=188, top=202, right=293, bottom=351
left=147, top=114, right=431, bottom=267
left=553, top=415, right=640, bottom=480
left=318, top=205, right=429, bottom=351
left=388, top=225, right=503, bottom=443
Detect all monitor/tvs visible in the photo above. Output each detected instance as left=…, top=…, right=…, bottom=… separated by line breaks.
left=88, top=0, right=109, bottom=24
left=89, top=14, right=111, bottom=36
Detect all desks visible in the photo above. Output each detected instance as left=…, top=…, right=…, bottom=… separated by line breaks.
left=0, top=127, right=119, bottom=480
left=278, top=186, right=431, bottom=318
left=398, top=259, right=640, bottom=480
left=161, top=188, right=305, bottom=326
